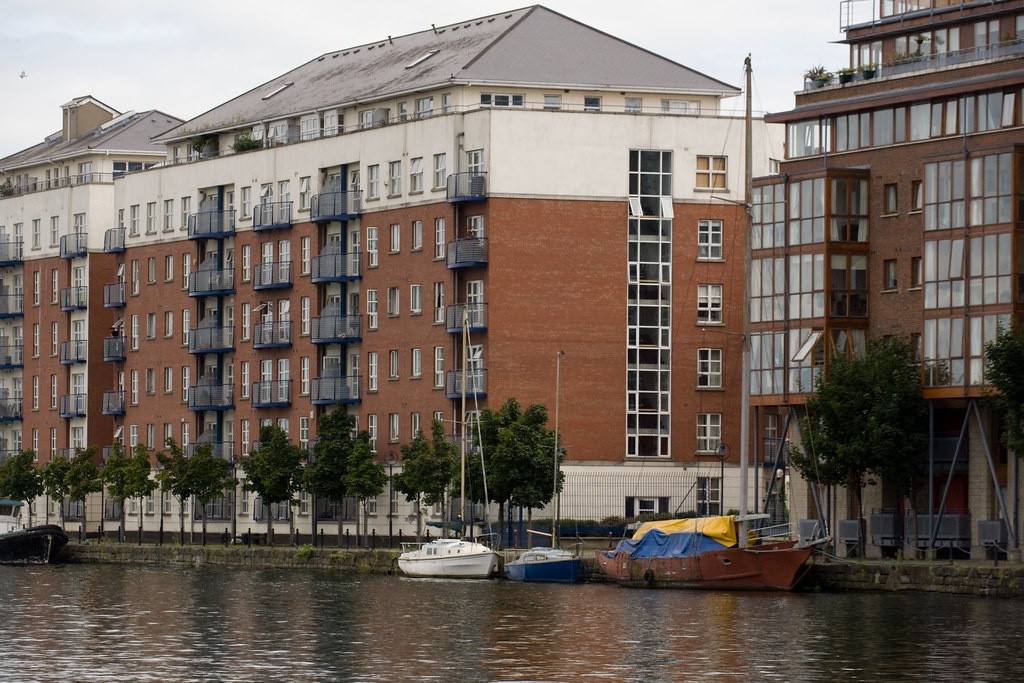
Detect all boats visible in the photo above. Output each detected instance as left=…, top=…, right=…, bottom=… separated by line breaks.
left=593, top=515, right=832, bottom=590
left=503, top=546, right=584, bottom=583
left=398, top=538, right=500, bottom=578
left=0, top=499, right=69, bottom=565
